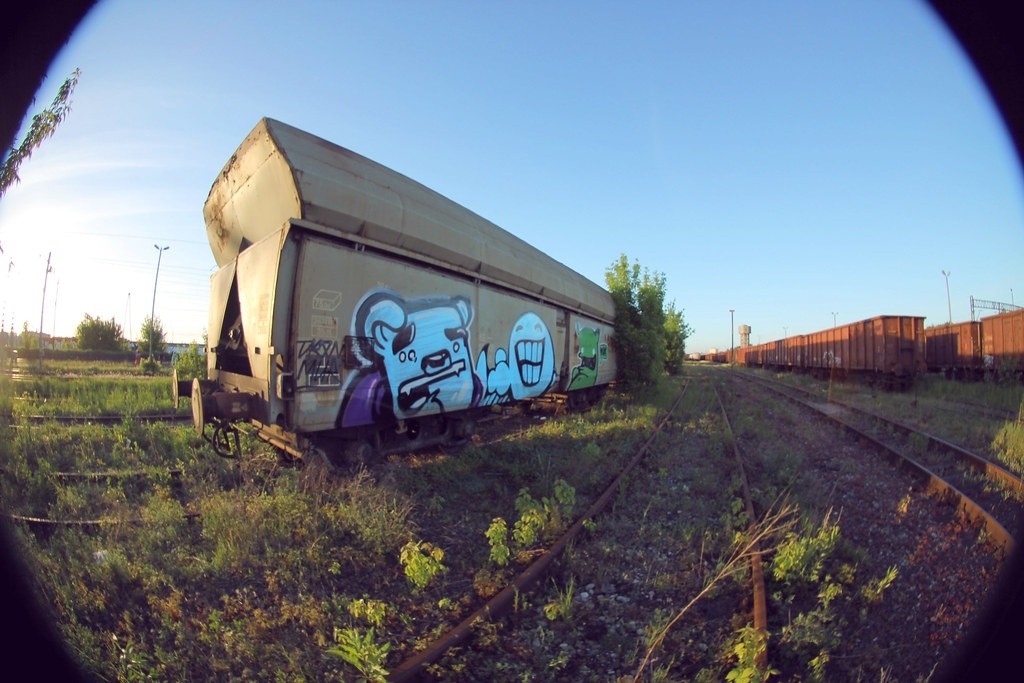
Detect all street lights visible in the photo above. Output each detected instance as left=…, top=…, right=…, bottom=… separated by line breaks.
left=149, top=245, right=169, bottom=357
left=832, top=312, right=838, bottom=326
left=729, top=310, right=735, bottom=376
left=942, top=270, right=951, bottom=322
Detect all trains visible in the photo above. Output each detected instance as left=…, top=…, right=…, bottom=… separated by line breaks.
left=701, top=315, right=926, bottom=392
left=173, top=118, right=616, bottom=470
left=924, top=310, right=1024, bottom=381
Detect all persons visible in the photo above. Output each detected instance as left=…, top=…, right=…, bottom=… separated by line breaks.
left=135, top=352, right=142, bottom=365
